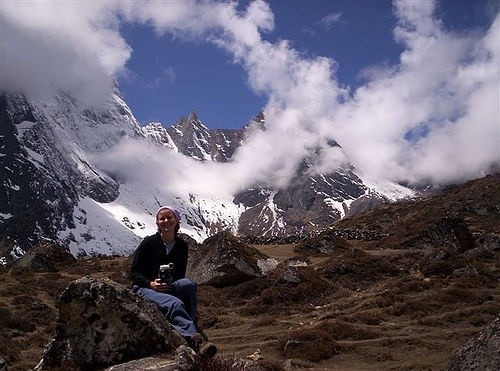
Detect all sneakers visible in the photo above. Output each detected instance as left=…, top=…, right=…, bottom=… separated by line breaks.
left=197, top=341, right=217, bottom=358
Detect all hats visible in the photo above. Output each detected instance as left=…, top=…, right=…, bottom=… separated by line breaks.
left=156, top=206, right=181, bottom=223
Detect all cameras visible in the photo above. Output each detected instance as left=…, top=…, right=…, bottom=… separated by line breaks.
left=159, top=262, right=174, bottom=279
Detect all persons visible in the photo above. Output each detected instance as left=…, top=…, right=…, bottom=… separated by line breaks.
left=127, top=204, right=216, bottom=365
